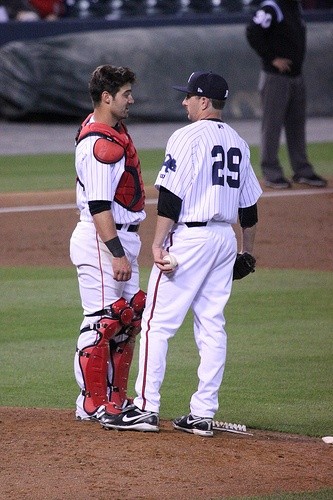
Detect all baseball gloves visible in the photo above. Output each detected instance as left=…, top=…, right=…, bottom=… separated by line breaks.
left=232, top=251, right=256, bottom=281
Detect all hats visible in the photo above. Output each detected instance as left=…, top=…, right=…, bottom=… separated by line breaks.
left=173, top=70, right=229, bottom=100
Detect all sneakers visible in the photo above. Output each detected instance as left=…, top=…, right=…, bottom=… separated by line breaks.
left=172, top=412, right=213, bottom=437
left=76, top=393, right=123, bottom=422
left=99, top=406, right=159, bottom=431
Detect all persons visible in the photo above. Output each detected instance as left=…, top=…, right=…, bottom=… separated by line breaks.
left=100, top=70, right=262, bottom=435
left=68, top=63, right=147, bottom=421
left=0, top=0, right=66, bottom=22
left=245, top=0, right=327, bottom=190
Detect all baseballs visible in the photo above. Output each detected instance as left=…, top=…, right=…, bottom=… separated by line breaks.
left=162, top=254, right=179, bottom=270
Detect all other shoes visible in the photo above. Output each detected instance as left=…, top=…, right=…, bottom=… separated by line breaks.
left=265, top=178, right=290, bottom=187
left=296, top=173, right=326, bottom=186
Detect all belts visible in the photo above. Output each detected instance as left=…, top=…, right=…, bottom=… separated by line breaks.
left=115, top=223, right=139, bottom=232
